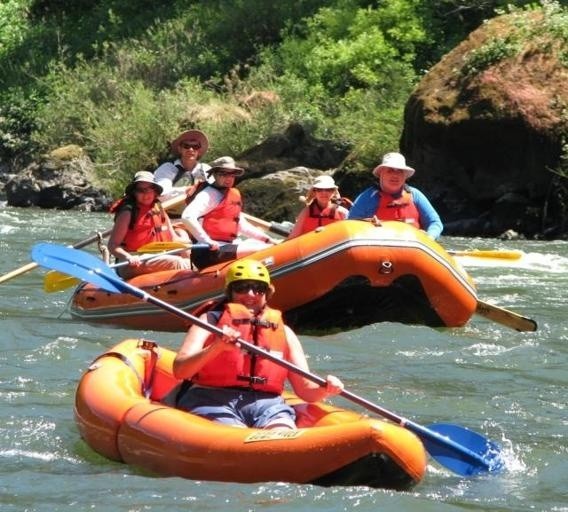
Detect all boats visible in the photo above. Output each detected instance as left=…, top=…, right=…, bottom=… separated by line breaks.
left=69, top=218, right=477, bottom=331
left=76, top=338, right=426, bottom=484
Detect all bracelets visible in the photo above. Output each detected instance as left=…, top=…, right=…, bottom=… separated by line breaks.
left=265, top=236, right=270, bottom=244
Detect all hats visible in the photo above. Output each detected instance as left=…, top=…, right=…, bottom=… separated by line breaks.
left=205, top=156, right=244, bottom=176
left=170, top=130, right=208, bottom=156
left=307, top=176, right=342, bottom=200
left=372, top=153, right=415, bottom=178
left=126, top=171, right=163, bottom=196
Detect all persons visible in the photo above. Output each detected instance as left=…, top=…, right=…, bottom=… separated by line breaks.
left=179, top=155, right=284, bottom=270
left=153, top=129, right=216, bottom=240
left=286, top=175, right=353, bottom=240
left=344, top=152, right=445, bottom=241
left=171, top=258, right=344, bottom=430
left=107, top=170, right=198, bottom=280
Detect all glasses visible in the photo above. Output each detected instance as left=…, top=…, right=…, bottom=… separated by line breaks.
left=219, top=171, right=235, bottom=178
left=136, top=184, right=155, bottom=194
left=181, top=144, right=200, bottom=151
left=228, top=281, right=268, bottom=295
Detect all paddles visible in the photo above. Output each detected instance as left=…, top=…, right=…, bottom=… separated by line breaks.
left=138, top=241, right=228, bottom=253
left=447, top=249, right=524, bottom=263
left=43, top=250, right=180, bottom=294
left=0, top=193, right=188, bottom=284
left=477, top=297, right=538, bottom=332
left=31, top=241, right=504, bottom=478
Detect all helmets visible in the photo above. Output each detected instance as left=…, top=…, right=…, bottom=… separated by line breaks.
left=224, top=260, right=270, bottom=295
